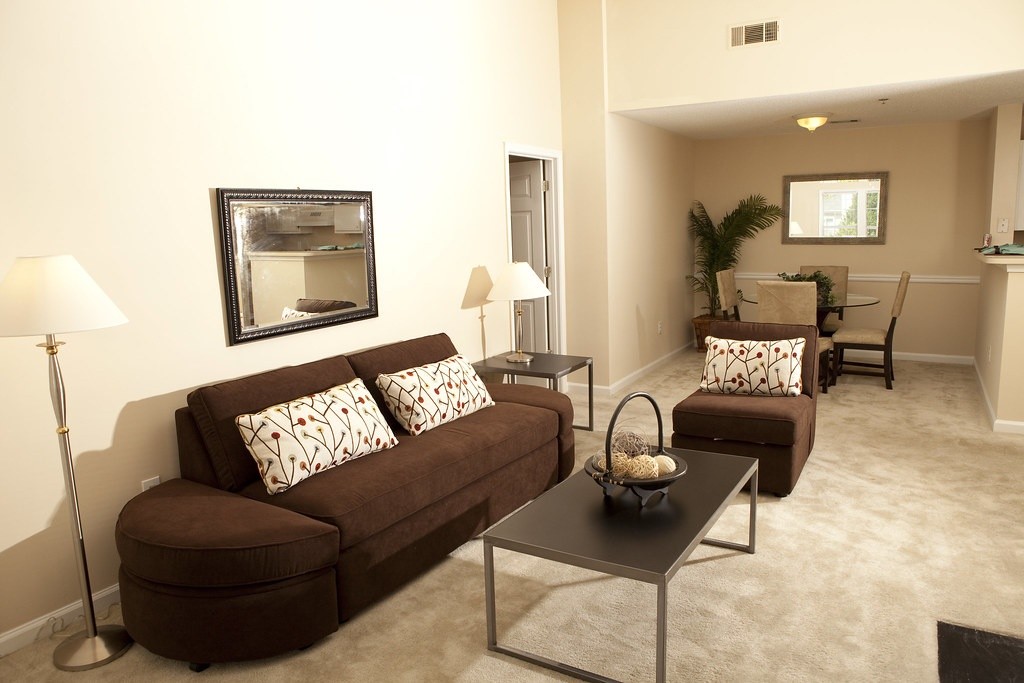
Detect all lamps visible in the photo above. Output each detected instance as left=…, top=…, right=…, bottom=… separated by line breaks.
left=792, top=111, right=830, bottom=134
left=0, top=252, right=133, bottom=672
left=485, top=260, right=552, bottom=362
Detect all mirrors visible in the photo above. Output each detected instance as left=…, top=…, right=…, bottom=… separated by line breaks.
left=216, top=187, right=378, bottom=346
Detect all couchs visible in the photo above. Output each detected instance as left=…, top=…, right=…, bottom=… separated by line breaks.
left=297, top=298, right=356, bottom=313
left=672, top=319, right=819, bottom=499
left=113, top=333, right=575, bottom=673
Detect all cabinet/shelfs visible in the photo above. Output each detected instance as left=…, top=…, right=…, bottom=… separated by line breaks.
left=263, top=201, right=365, bottom=235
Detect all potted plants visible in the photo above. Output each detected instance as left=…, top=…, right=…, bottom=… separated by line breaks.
left=683, top=192, right=789, bottom=351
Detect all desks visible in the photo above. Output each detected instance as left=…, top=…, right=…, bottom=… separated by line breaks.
left=471, top=349, right=595, bottom=432
left=740, top=293, right=880, bottom=374
left=483, top=444, right=759, bottom=683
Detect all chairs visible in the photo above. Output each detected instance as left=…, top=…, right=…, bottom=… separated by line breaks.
left=831, top=270, right=911, bottom=390
left=715, top=265, right=849, bottom=393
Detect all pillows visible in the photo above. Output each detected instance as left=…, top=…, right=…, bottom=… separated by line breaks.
left=233, top=376, right=399, bottom=495
left=698, top=335, right=806, bottom=397
left=280, top=306, right=318, bottom=321
left=374, top=352, right=496, bottom=437
